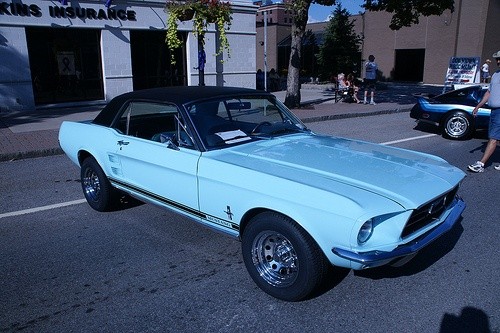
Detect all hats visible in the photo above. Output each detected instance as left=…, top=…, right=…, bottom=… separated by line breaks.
left=485, top=59, right=491, bottom=63
left=492, top=51, right=500, bottom=58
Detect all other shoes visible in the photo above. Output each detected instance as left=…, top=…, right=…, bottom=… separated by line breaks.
left=369, top=101, right=376, bottom=104
left=363, top=101, right=369, bottom=104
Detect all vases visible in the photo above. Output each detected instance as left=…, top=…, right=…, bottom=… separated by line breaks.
left=176, top=9, right=195, bottom=21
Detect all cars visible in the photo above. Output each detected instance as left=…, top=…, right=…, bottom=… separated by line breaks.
left=57, top=85, right=468, bottom=302
left=410, top=85, right=493, bottom=141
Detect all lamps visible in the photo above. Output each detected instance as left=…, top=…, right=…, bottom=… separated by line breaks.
left=105, top=0, right=113, bottom=7
left=62, top=0, right=67, bottom=5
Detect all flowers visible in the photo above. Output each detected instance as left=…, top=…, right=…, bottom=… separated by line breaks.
left=163, top=0, right=233, bottom=65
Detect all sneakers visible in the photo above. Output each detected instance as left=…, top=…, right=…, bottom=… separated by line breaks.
left=494, top=165, right=500, bottom=170
left=467, top=161, right=484, bottom=173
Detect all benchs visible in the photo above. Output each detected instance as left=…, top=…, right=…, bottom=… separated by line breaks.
left=333, top=78, right=356, bottom=103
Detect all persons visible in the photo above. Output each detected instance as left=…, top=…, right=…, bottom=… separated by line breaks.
left=467, top=51, right=500, bottom=172
left=364, top=55, right=378, bottom=105
left=256, top=69, right=286, bottom=91
left=300, top=72, right=319, bottom=83
left=339, top=73, right=361, bottom=103
left=481, top=59, right=491, bottom=83
